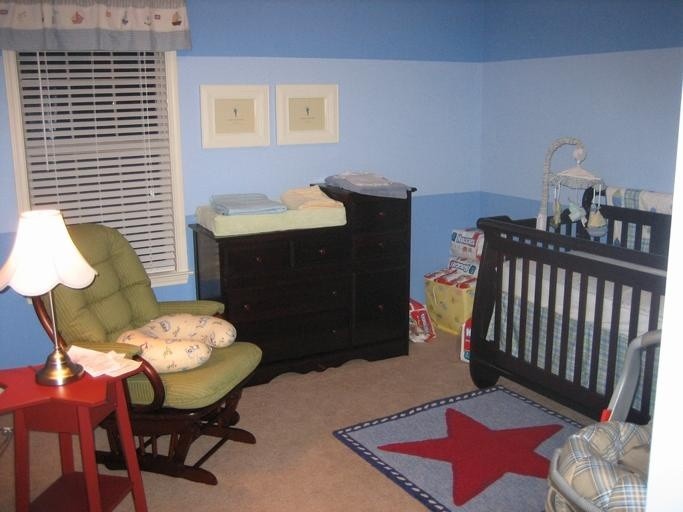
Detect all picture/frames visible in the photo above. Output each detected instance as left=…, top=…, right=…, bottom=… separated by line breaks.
left=273, top=81, right=340, bottom=148
left=192, top=81, right=271, bottom=149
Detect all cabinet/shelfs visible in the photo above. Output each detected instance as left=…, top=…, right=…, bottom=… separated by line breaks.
left=306, top=179, right=416, bottom=361
left=187, top=176, right=351, bottom=389
left=0, top=359, right=149, bottom=512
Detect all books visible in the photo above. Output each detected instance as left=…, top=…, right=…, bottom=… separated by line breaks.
left=66, top=345, right=143, bottom=378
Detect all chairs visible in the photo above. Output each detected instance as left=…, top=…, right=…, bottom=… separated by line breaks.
left=546, top=329, right=663, bottom=512
left=31, top=221, right=267, bottom=488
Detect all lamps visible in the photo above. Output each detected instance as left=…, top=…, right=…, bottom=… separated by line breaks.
left=0, top=206, right=103, bottom=392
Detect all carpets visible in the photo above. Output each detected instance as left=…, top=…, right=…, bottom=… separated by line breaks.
left=329, top=384, right=590, bottom=512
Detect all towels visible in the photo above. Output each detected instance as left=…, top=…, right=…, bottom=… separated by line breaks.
left=204, top=190, right=288, bottom=217
left=321, top=168, right=413, bottom=201
left=279, top=185, right=344, bottom=212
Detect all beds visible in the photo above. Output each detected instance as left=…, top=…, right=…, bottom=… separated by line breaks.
left=467, top=180, right=674, bottom=424
